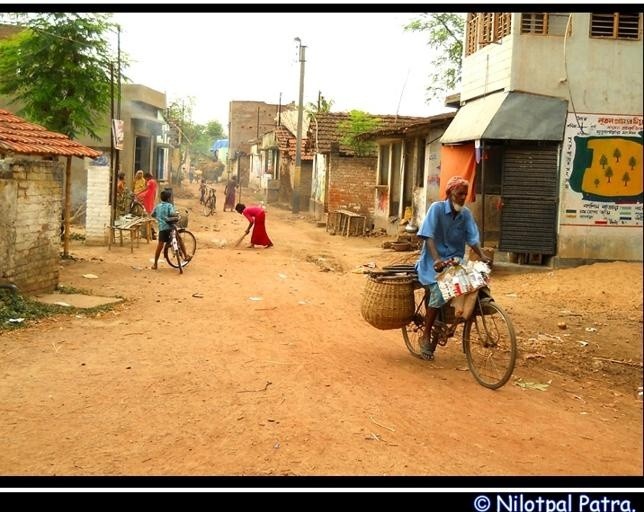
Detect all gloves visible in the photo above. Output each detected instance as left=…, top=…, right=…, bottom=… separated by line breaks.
left=359, top=273, right=415, bottom=330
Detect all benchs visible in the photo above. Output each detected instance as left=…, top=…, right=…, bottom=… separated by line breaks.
left=326, top=209, right=367, bottom=237
left=107, top=216, right=156, bottom=253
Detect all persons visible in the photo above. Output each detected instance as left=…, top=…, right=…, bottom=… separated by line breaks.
left=235, top=203, right=273, bottom=248
left=413, top=175, right=494, bottom=362
left=150, top=190, right=194, bottom=270
left=116, top=172, right=127, bottom=215
left=224, top=175, right=239, bottom=212
left=130, top=169, right=147, bottom=195
left=182, top=164, right=196, bottom=183
left=199, top=178, right=207, bottom=205
left=135, top=173, right=159, bottom=214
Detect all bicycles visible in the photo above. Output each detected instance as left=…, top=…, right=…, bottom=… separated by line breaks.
left=402, top=257, right=518, bottom=390
left=118, top=187, right=148, bottom=230
left=202, top=186, right=217, bottom=217
left=163, top=209, right=197, bottom=275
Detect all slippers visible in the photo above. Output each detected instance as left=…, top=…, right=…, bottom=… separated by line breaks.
left=420, top=339, right=437, bottom=363
